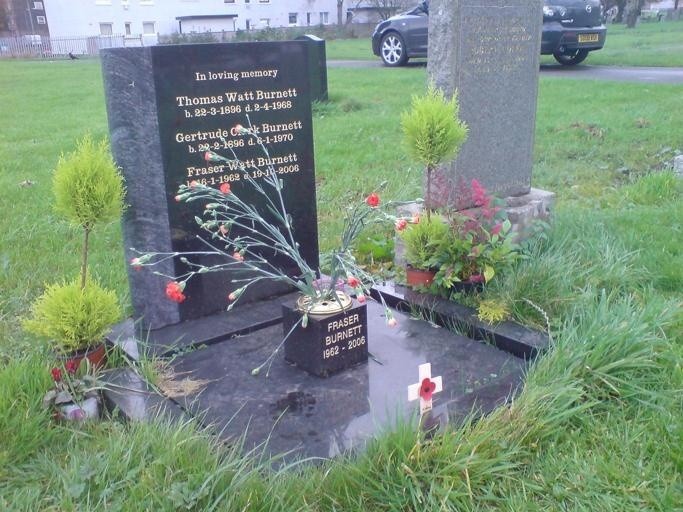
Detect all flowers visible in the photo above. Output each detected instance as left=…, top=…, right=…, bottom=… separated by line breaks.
left=43, top=357, right=122, bottom=404
left=446, top=178, right=514, bottom=273
left=124, top=114, right=424, bottom=374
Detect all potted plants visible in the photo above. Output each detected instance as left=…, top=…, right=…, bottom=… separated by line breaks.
left=404, top=214, right=454, bottom=293
left=18, top=278, right=128, bottom=371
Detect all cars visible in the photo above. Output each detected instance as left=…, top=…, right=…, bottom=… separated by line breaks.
left=372, top=0, right=608, bottom=66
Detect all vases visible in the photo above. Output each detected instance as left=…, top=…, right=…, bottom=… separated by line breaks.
left=56, top=396, right=100, bottom=423
left=452, top=273, right=486, bottom=294
